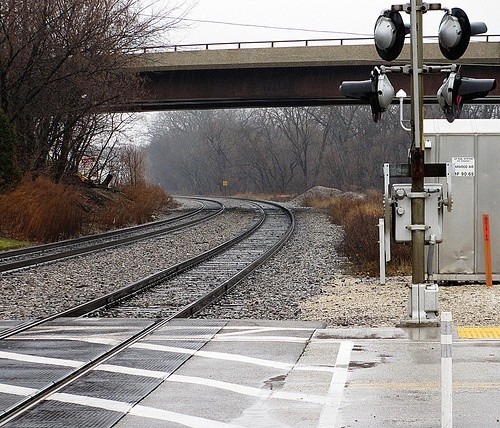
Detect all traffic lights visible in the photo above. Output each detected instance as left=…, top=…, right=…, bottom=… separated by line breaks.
left=336, top=71, right=395, bottom=123
left=373, top=10, right=407, bottom=61
left=435, top=72, right=495, bottom=123
left=441, top=7, right=488, bottom=61
